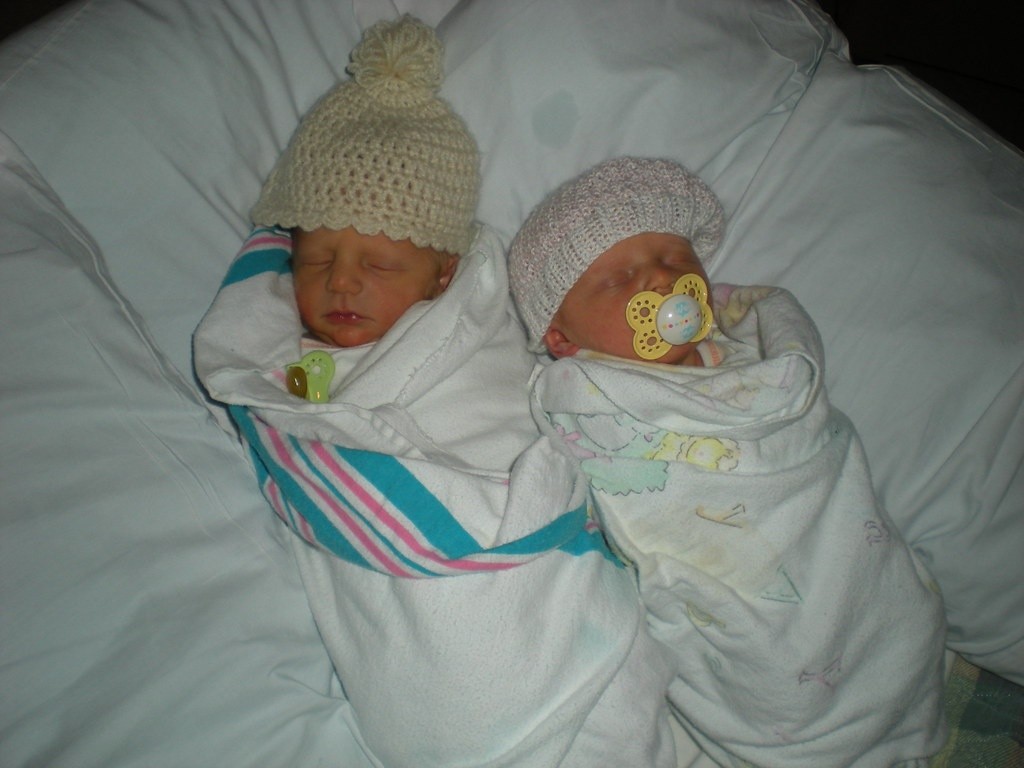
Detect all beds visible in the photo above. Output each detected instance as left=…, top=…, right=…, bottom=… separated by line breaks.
left=0, top=0, right=1024, bottom=768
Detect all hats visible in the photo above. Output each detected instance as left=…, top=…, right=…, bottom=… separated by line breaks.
left=506, top=156, right=724, bottom=355
left=252, top=13, right=482, bottom=256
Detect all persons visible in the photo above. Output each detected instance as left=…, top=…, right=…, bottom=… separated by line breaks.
left=192, top=15, right=681, bottom=768
left=506, top=157, right=946, bottom=768
